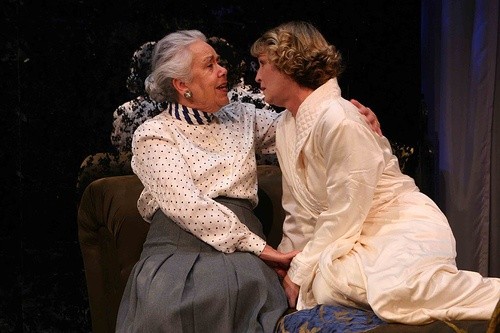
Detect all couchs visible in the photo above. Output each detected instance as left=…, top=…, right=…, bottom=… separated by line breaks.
left=77, top=166, right=286, bottom=333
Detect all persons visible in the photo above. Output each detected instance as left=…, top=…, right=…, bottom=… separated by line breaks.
left=113, top=30, right=382, bottom=332
left=111, top=34, right=282, bottom=166
left=250, top=21, right=500, bottom=325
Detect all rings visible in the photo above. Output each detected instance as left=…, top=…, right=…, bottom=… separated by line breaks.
left=372, top=121, right=380, bottom=126
